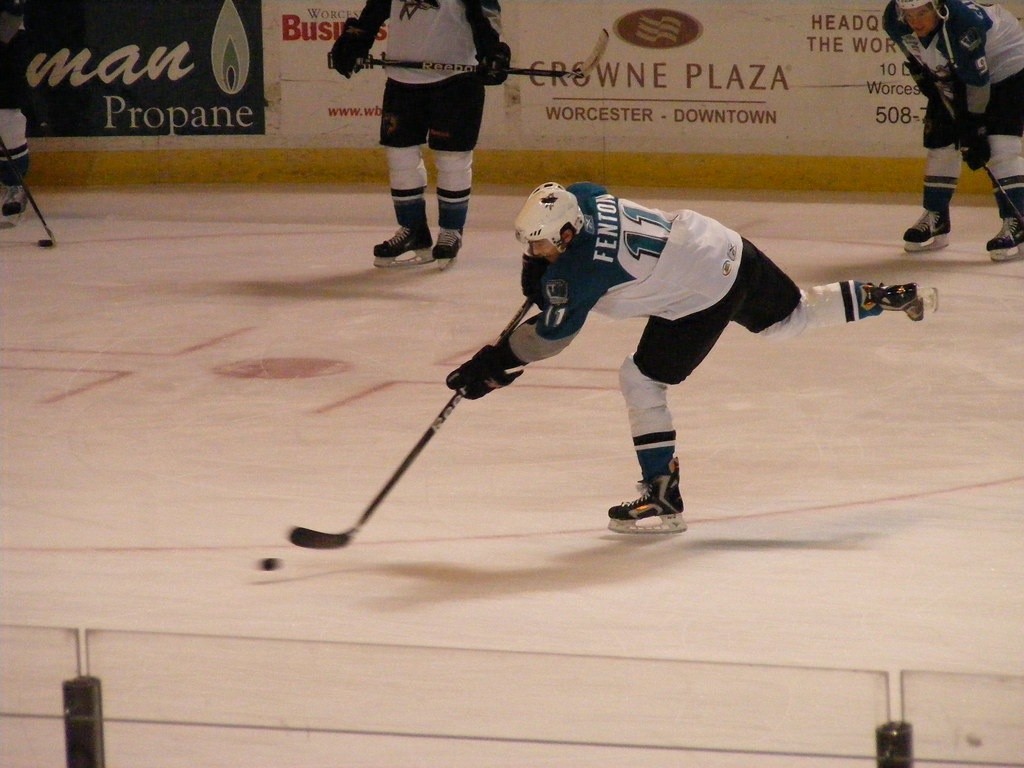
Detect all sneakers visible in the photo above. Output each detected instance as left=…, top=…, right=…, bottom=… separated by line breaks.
left=373, top=225, right=434, bottom=267
left=903, top=205, right=952, bottom=253
left=860, top=282, right=939, bottom=322
left=607, top=456, right=688, bottom=534
left=3, top=185, right=28, bottom=226
left=986, top=214, right=1024, bottom=262
left=431, top=226, right=463, bottom=271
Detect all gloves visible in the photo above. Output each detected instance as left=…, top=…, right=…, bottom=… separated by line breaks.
left=904, top=60, right=941, bottom=105
left=954, top=111, right=992, bottom=171
left=476, top=42, right=511, bottom=87
left=446, top=338, right=528, bottom=399
left=330, top=17, right=376, bottom=80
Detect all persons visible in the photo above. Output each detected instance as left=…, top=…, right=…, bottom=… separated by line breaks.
left=881, top=0, right=1024, bottom=262
left=446, top=182, right=939, bottom=535
left=0, top=0, right=29, bottom=225
left=331, top=0, right=511, bottom=272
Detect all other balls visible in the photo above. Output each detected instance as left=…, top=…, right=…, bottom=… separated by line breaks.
left=38, top=239, right=54, bottom=247
left=261, top=558, right=279, bottom=570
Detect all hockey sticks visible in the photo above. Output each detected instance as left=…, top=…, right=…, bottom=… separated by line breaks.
left=940, top=92, right=1024, bottom=231
left=288, top=296, right=536, bottom=552
left=0, top=133, right=58, bottom=248
left=361, top=27, right=613, bottom=79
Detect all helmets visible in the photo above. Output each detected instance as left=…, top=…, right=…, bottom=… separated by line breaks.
left=896, top=0, right=934, bottom=10
left=515, top=182, right=585, bottom=245
